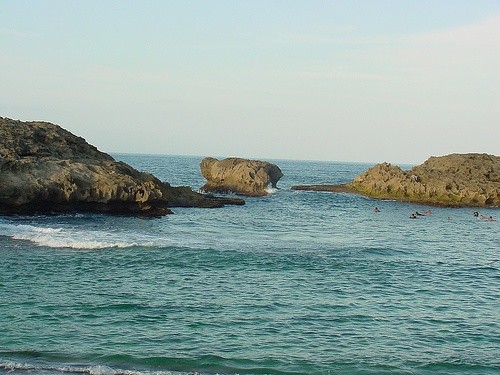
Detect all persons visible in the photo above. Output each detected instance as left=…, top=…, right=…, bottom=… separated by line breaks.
left=415, top=211, right=422, bottom=216
left=472, top=211, right=494, bottom=222
left=410, top=213, right=416, bottom=218
left=374, top=206, right=380, bottom=212
